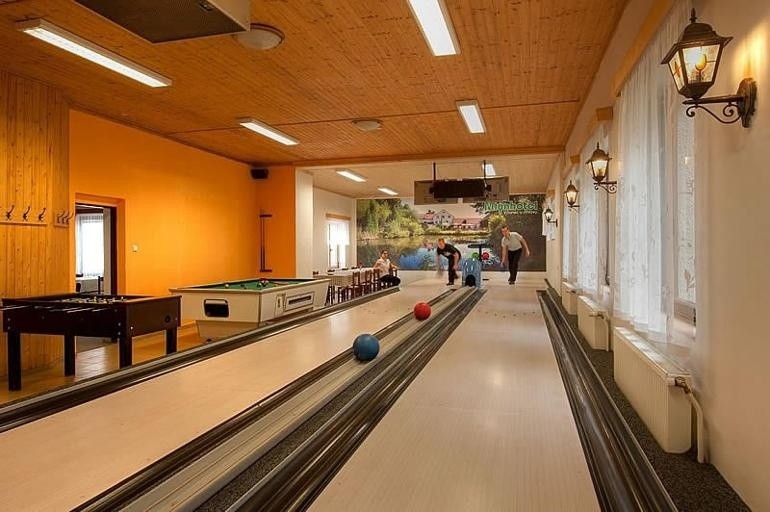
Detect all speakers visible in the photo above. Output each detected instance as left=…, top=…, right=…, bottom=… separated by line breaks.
left=251, top=169, right=267, bottom=179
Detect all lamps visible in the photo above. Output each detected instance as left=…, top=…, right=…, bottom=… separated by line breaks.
left=660, top=6, right=756, bottom=131
left=233, top=24, right=285, bottom=50
left=336, top=168, right=367, bottom=183
left=406, top=0, right=487, bottom=134
left=236, top=117, right=300, bottom=146
left=13, top=19, right=173, bottom=88
left=544, top=141, right=616, bottom=228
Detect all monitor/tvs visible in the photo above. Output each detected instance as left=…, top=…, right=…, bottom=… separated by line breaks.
left=414, top=179, right=458, bottom=205
left=462, top=176, right=509, bottom=203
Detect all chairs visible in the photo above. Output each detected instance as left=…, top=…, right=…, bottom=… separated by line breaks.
left=313, top=267, right=380, bottom=305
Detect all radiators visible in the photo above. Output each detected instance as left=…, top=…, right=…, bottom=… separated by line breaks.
left=560, top=281, right=704, bottom=465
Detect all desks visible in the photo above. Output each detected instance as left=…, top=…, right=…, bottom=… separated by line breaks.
left=169, top=277, right=332, bottom=342
left=0, top=292, right=182, bottom=393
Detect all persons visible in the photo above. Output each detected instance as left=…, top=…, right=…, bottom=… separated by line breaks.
left=434, top=237, right=462, bottom=286
left=498, top=226, right=530, bottom=285
left=372, top=249, right=401, bottom=287
